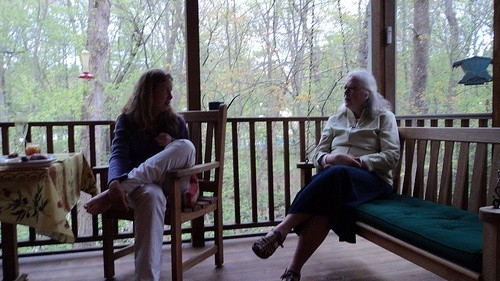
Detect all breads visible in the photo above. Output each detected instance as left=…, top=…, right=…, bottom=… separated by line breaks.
left=7, top=152, right=18, bottom=158
left=26, top=145, right=41, bottom=155
left=21, top=153, right=47, bottom=160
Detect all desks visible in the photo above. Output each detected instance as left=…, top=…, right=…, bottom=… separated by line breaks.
left=0, top=153, right=98, bottom=281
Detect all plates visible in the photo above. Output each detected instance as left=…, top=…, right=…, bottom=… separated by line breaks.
left=0, top=154, right=56, bottom=166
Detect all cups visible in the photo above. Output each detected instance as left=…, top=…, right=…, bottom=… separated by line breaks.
left=25, top=142, right=42, bottom=156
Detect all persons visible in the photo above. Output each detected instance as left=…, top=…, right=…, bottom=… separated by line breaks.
left=84, top=69, right=196, bottom=281
left=252, top=69, right=399, bottom=281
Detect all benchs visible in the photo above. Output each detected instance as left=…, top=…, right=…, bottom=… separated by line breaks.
left=296, top=125, right=500, bottom=281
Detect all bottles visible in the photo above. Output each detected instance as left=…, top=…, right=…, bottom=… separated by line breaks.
left=15, top=119, right=30, bottom=157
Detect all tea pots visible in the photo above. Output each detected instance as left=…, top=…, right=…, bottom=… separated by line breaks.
left=202, top=89, right=241, bottom=111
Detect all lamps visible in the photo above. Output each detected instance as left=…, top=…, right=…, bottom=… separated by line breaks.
left=78, top=50, right=95, bottom=78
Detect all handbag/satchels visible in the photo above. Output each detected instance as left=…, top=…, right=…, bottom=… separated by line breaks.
left=184, top=174, right=199, bottom=208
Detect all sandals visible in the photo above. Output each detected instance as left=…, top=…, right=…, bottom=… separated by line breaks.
left=252, top=229, right=284, bottom=259
left=280, top=268, right=300, bottom=281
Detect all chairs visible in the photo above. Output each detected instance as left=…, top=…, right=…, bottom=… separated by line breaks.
left=92, top=102, right=228, bottom=281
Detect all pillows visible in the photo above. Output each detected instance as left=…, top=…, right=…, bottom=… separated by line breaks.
left=182, top=175, right=200, bottom=211
